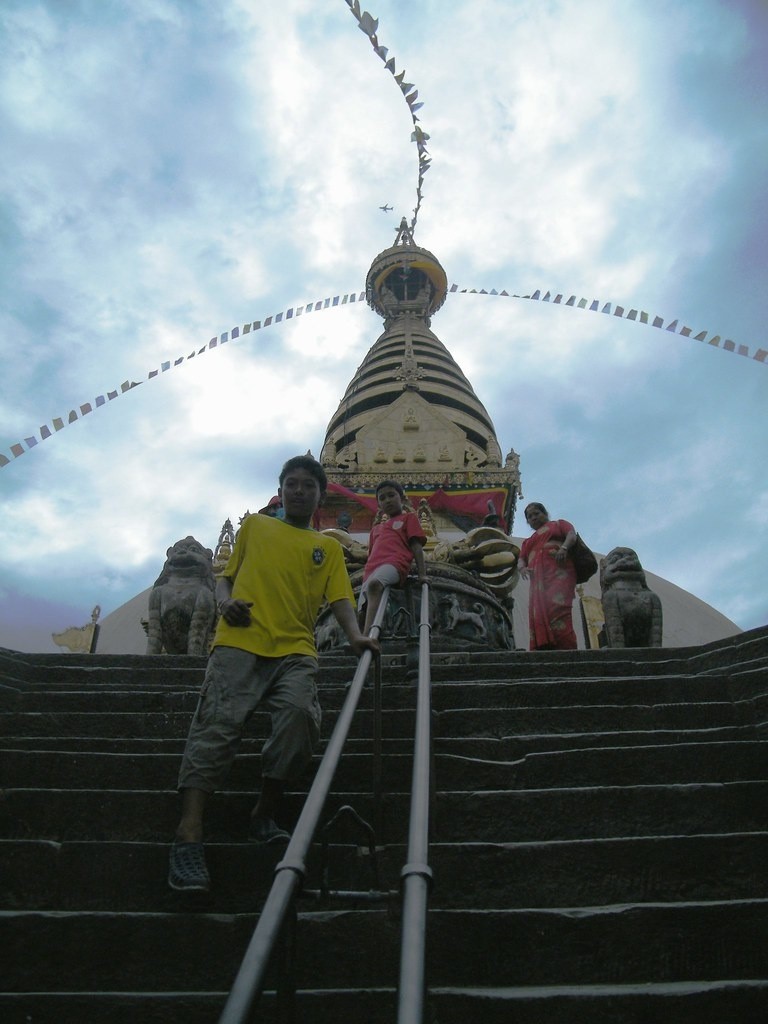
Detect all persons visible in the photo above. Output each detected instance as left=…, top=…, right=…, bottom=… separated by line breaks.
left=357, top=480, right=433, bottom=638
left=518, top=504, right=598, bottom=651
left=168, top=457, right=383, bottom=892
left=405, top=408, right=417, bottom=424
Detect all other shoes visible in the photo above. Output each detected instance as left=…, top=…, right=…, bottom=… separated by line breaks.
left=248, top=826, right=291, bottom=844
left=167, top=828, right=210, bottom=891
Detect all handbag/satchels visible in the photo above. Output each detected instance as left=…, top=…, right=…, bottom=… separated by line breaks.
left=557, top=520, right=598, bottom=584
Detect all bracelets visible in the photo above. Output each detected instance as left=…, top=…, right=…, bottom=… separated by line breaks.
left=216, top=598, right=230, bottom=611
left=560, top=546, right=569, bottom=552
